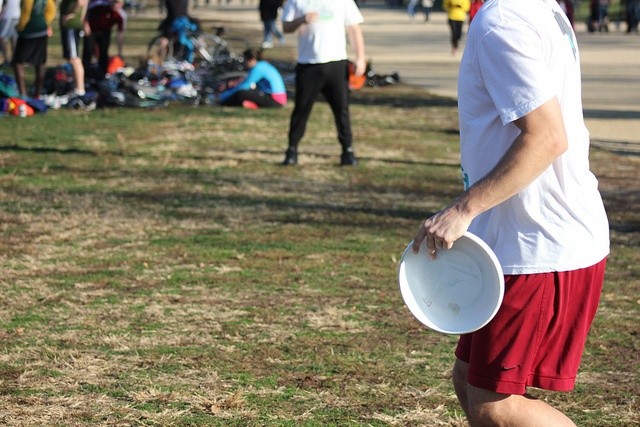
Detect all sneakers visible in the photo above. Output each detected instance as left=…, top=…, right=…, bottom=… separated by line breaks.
left=341, top=149, right=356, bottom=165
left=284, top=148, right=297, bottom=165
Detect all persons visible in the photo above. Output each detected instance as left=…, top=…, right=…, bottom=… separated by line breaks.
left=421, top=0, right=435, bottom=23
left=442, top=0, right=470, bottom=55
left=281, top=0, right=367, bottom=169
left=204, top=47, right=287, bottom=110
left=258, top=1, right=287, bottom=49
left=412, top=0, right=611, bottom=427
left=1, top=0, right=203, bottom=102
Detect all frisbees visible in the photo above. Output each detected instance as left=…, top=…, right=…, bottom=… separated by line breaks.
left=397, top=230, right=504, bottom=333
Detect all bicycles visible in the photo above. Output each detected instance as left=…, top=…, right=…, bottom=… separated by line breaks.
left=147, top=25, right=243, bottom=65
